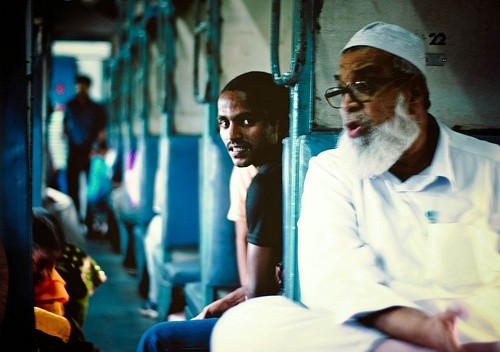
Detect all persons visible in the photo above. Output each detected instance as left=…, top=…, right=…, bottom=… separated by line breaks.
left=211, top=22, right=500, bottom=352
left=29, top=76, right=162, bottom=352
left=137, top=72, right=290, bottom=352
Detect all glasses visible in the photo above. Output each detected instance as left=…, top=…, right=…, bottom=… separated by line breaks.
left=324, top=75, right=402, bottom=110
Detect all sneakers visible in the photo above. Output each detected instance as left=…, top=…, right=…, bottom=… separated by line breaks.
left=137, top=303, right=160, bottom=319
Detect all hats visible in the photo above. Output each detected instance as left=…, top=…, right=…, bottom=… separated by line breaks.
left=340, top=20, right=428, bottom=79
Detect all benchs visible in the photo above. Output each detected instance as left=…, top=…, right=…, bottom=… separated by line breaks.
left=104, top=133, right=342, bottom=321
